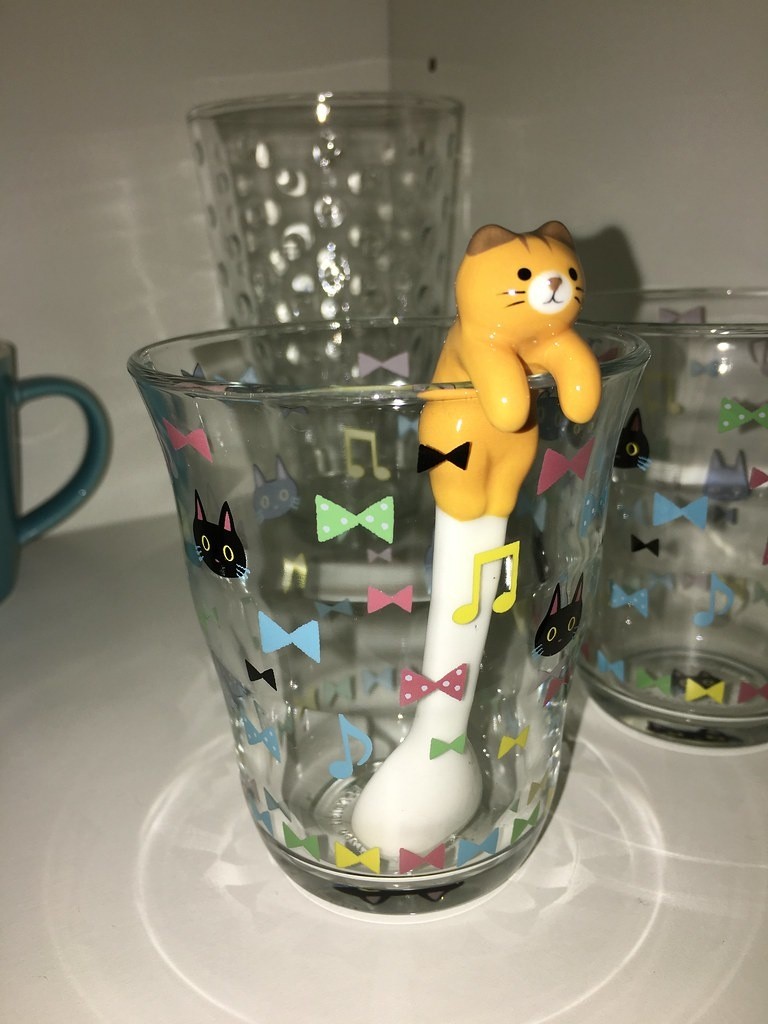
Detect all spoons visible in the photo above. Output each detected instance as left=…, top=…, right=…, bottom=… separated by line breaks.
left=353, top=223, right=601, bottom=854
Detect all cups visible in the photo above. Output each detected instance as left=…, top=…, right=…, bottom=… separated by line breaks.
left=185, top=88, right=467, bottom=329
left=1, top=340, right=112, bottom=605
left=125, top=317, right=652, bottom=915
left=575, top=286, right=767, bottom=748
